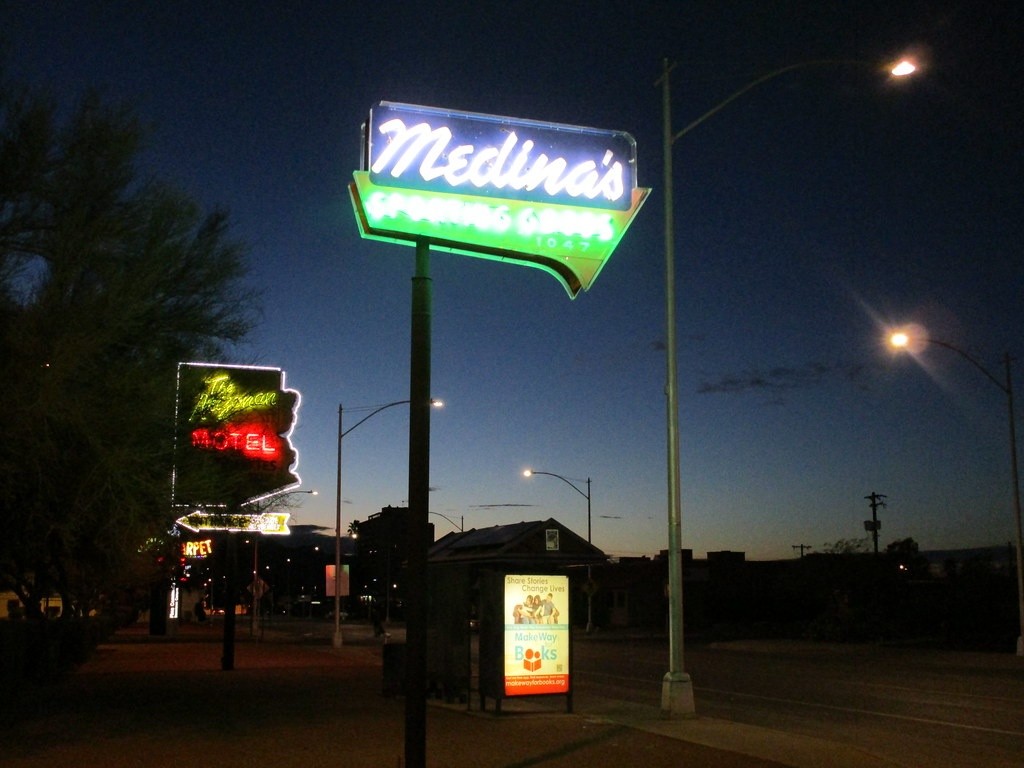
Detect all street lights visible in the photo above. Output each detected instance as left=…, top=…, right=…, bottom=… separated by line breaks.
left=523, top=467, right=595, bottom=636
left=331, top=396, right=446, bottom=649
left=657, top=41, right=927, bottom=720
left=882, top=320, right=1023, bottom=659
left=250, top=489, right=320, bottom=638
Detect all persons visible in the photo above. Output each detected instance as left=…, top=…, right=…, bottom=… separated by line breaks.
left=518, top=593, right=556, bottom=626
left=195, top=600, right=205, bottom=622
left=369, top=614, right=385, bottom=637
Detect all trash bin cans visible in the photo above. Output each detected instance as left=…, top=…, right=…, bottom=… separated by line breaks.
left=382, top=643, right=410, bottom=699
